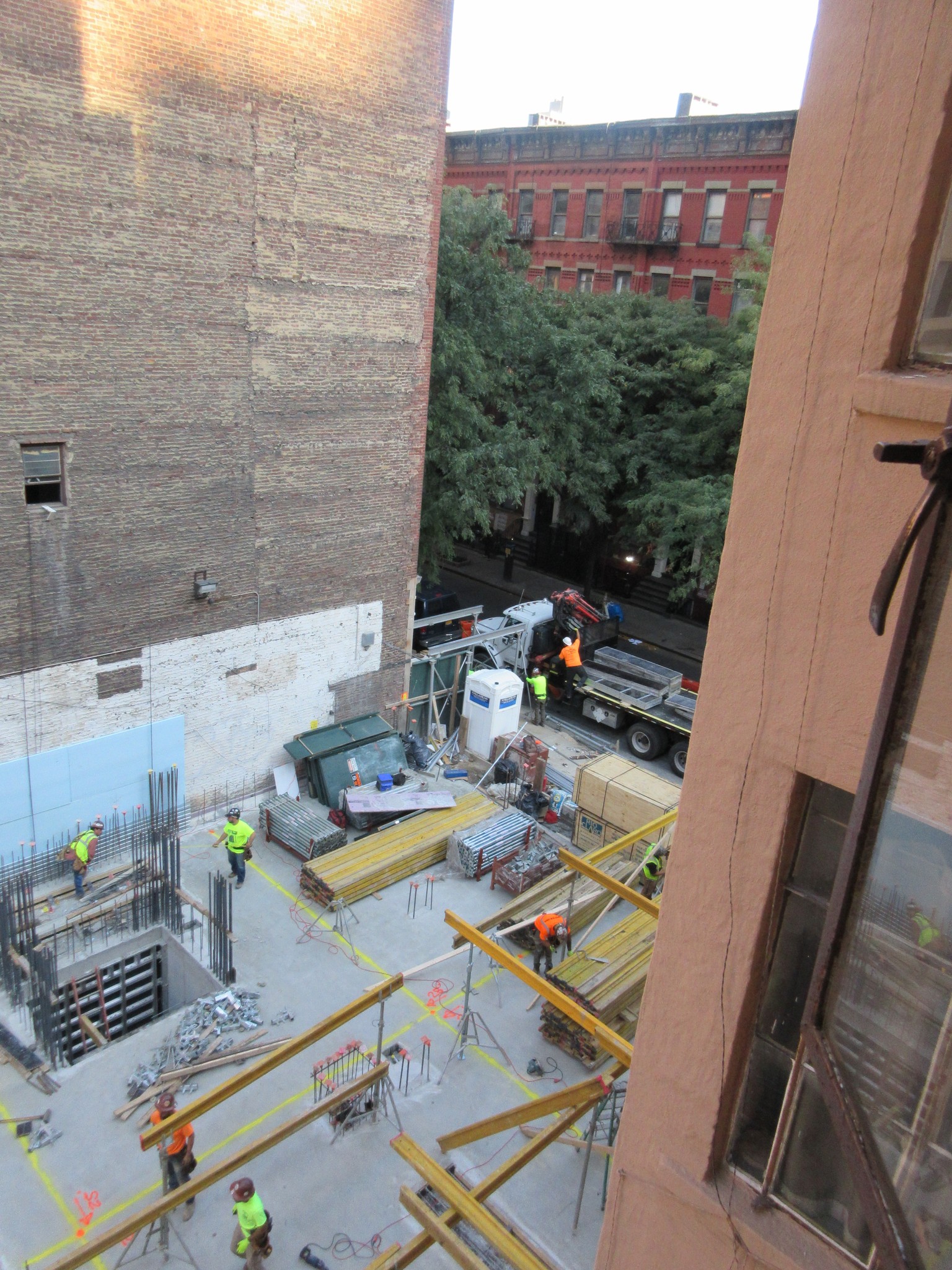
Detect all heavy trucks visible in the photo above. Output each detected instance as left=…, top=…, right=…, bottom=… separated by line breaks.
left=471, top=587, right=701, bottom=779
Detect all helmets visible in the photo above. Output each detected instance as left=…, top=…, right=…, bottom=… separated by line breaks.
left=155, top=1092, right=178, bottom=1111
left=557, top=926, right=567, bottom=941
left=519, top=736, right=527, bottom=748
left=89, top=821, right=105, bottom=829
left=563, top=637, right=572, bottom=646
left=230, top=1177, right=255, bottom=1201
left=224, top=808, right=240, bottom=817
left=532, top=667, right=541, bottom=675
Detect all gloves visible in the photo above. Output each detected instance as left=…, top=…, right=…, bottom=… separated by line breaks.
left=236, top=1238, right=250, bottom=1254
left=232, top=1205, right=237, bottom=1215
left=550, top=945, right=556, bottom=952
left=574, top=628, right=578, bottom=631
left=567, top=950, right=574, bottom=956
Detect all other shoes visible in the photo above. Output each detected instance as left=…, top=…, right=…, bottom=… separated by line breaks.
left=183, top=1201, right=195, bottom=1221
left=532, top=720, right=544, bottom=727
left=533, top=962, right=553, bottom=975
left=228, top=871, right=242, bottom=889
left=567, top=687, right=584, bottom=698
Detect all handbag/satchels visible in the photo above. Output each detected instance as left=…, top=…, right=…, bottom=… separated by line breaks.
left=529, top=922, right=537, bottom=935
left=180, top=1145, right=197, bottom=1173
left=57, top=847, right=77, bottom=862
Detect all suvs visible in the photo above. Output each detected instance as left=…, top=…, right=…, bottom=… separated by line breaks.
left=414, top=578, right=464, bottom=650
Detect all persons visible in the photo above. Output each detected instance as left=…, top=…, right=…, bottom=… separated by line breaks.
left=150, top=1092, right=196, bottom=1222
left=524, top=667, right=547, bottom=727
left=532, top=914, right=573, bottom=974
left=559, top=628, right=588, bottom=698
left=904, top=901, right=944, bottom=972
left=212, top=808, right=256, bottom=889
left=637, top=843, right=670, bottom=909
left=67, top=821, right=105, bottom=900
left=229, top=1177, right=272, bottom=1270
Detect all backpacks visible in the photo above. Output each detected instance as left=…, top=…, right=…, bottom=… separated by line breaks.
left=392, top=773, right=406, bottom=785
left=523, top=735, right=538, bottom=754
left=515, top=784, right=540, bottom=822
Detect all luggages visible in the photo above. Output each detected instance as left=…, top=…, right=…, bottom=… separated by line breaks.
left=494, top=758, right=515, bottom=784
left=327, top=788, right=348, bottom=829
